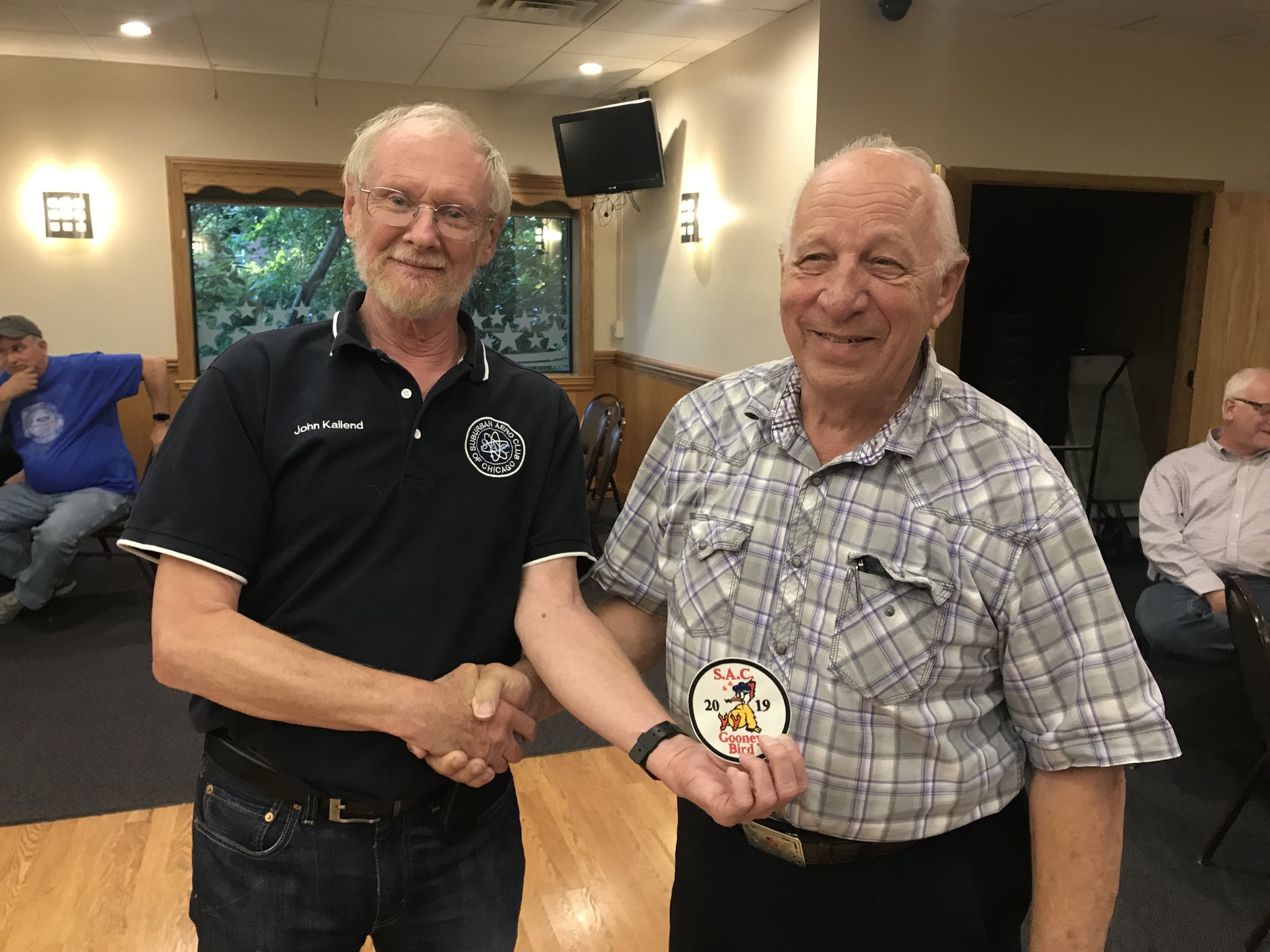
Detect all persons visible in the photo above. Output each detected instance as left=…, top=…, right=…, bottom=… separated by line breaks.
left=1134, top=366, right=1270, bottom=664
left=401, top=135, right=1183, bottom=952
left=0, top=316, right=172, bottom=622
left=115, top=101, right=811, bottom=952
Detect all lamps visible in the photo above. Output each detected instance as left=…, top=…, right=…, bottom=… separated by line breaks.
left=42, top=190, right=93, bottom=239
left=680, top=192, right=700, bottom=243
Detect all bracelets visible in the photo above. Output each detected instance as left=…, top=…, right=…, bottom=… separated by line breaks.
left=153, top=412, right=170, bottom=421
left=628, top=720, right=693, bottom=782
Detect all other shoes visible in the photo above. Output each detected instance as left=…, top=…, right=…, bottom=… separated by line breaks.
left=0, top=593, right=27, bottom=625
left=51, top=577, right=77, bottom=596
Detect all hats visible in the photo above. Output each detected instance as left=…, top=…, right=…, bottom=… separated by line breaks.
left=0, top=315, right=42, bottom=340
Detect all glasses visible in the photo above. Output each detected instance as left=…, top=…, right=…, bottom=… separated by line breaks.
left=1234, top=396, right=1270, bottom=416
left=361, top=186, right=495, bottom=243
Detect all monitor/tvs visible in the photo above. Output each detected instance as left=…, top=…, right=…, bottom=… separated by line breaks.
left=552, top=98, right=666, bottom=198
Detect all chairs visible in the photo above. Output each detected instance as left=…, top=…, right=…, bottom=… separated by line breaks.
left=1198, top=573, right=1270, bottom=952
left=580, top=391, right=627, bottom=559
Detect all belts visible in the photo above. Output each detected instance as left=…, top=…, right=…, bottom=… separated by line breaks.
left=739, top=818, right=868, bottom=871
left=205, top=732, right=447, bottom=825
